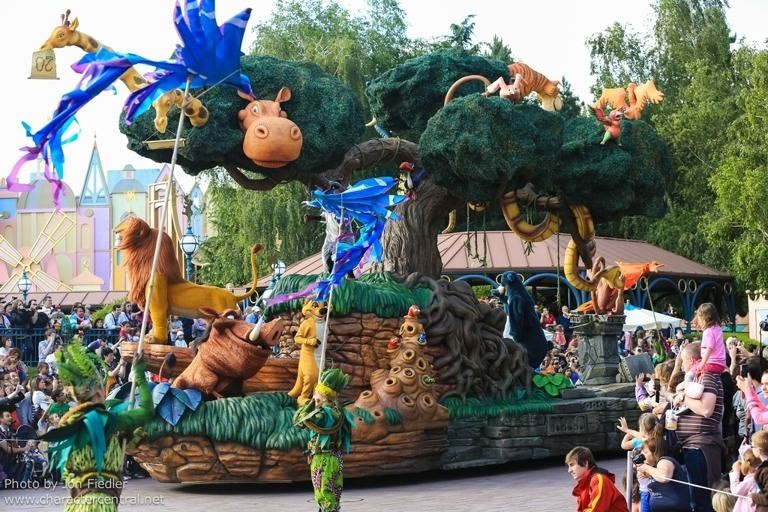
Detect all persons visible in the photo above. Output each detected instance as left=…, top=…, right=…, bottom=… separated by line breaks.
left=289, top=385, right=356, bottom=511
left=40, top=346, right=159, bottom=509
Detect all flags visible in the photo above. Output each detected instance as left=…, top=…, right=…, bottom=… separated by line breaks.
left=112, top=217, right=335, bottom=407
left=145, top=85, right=213, bottom=135
left=237, top=86, right=304, bottom=171
left=484, top=60, right=668, bottom=149
left=41, top=9, right=155, bottom=97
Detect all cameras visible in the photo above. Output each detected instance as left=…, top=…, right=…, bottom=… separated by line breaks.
left=18, top=385, right=25, bottom=390
left=738, top=364, right=748, bottom=378
left=572, top=359, right=577, bottom=363
left=36, top=306, right=42, bottom=310
left=55, top=335, right=61, bottom=339
left=654, top=379, right=660, bottom=389
left=632, top=452, right=646, bottom=465
left=52, top=376, right=56, bottom=379
left=540, top=360, right=548, bottom=368
left=643, top=375, right=651, bottom=382
